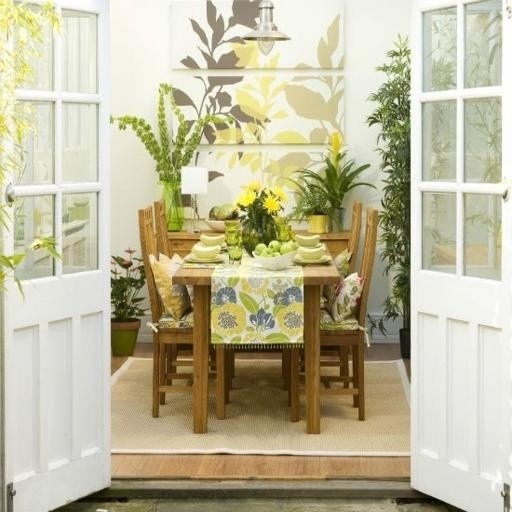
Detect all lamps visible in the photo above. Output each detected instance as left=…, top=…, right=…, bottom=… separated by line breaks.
left=243, top=0, right=291, bottom=55
left=181, top=166, right=209, bottom=233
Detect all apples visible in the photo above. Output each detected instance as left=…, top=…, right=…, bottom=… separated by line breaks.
left=255, top=240, right=298, bottom=258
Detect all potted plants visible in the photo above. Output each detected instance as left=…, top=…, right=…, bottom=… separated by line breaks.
left=285, top=175, right=334, bottom=234
left=366, top=59, right=448, bottom=357
left=108, top=84, right=234, bottom=232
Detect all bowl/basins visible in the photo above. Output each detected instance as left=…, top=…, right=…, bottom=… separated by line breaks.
left=206, top=219, right=238, bottom=232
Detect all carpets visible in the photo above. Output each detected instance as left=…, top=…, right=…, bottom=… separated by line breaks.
left=111, top=357, right=410, bottom=457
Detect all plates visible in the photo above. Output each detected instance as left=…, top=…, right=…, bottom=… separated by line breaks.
left=187, top=240, right=332, bottom=270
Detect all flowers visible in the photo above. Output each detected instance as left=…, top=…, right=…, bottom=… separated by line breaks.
left=298, top=132, right=377, bottom=207
left=110, top=247, right=151, bottom=321
left=238, top=183, right=289, bottom=236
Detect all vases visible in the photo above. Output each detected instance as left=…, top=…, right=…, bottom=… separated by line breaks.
left=330, top=209, right=345, bottom=234
left=111, top=318, right=141, bottom=356
